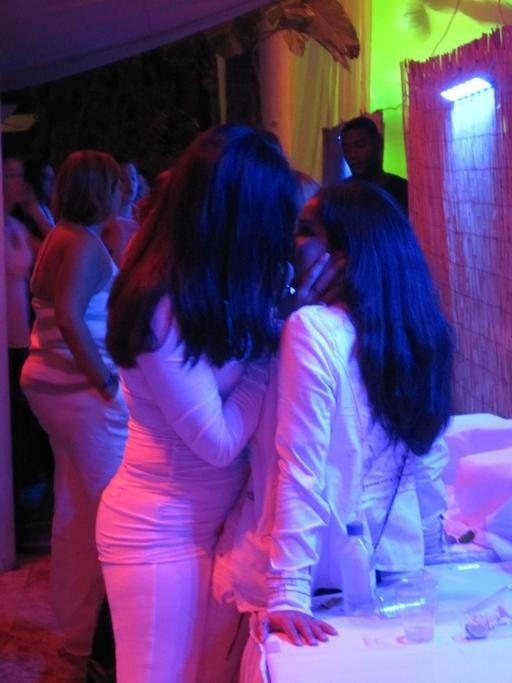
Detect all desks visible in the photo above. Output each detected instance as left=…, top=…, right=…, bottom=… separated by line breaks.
left=239, top=559, right=512, bottom=683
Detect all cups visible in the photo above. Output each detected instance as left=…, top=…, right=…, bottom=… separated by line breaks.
left=393, top=576, right=440, bottom=644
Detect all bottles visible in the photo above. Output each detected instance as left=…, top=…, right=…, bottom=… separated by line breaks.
left=340, top=521, right=376, bottom=618
